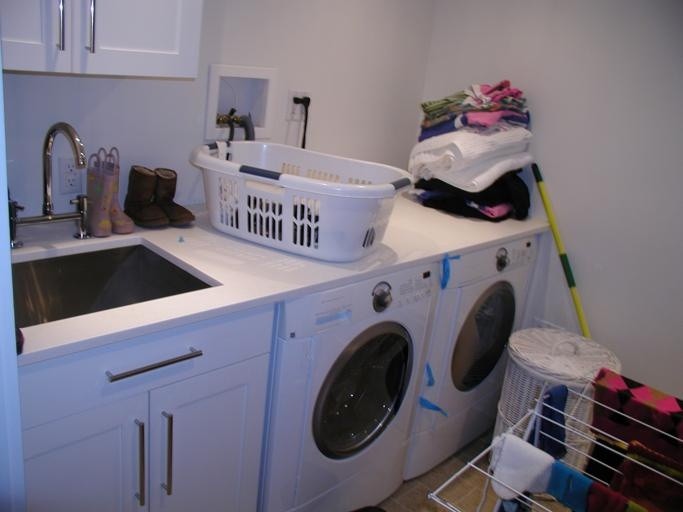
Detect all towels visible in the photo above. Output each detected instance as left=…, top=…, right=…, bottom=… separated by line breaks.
left=485, top=367, right=682, bottom=512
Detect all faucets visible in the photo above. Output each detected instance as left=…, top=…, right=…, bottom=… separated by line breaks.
left=42, top=122, right=87, bottom=216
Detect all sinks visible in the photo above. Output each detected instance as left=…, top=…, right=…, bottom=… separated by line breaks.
left=11, top=236, right=225, bottom=329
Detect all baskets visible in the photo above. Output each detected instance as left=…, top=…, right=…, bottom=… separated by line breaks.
left=189, top=141, right=414, bottom=262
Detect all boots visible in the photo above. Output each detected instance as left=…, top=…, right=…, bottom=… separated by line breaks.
left=125, top=166, right=195, bottom=228
left=87, top=148, right=134, bottom=237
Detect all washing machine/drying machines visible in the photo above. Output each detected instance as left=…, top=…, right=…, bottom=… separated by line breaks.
left=190, top=210, right=448, bottom=511
left=389, top=182, right=554, bottom=482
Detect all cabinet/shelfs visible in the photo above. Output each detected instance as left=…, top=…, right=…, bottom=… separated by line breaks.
left=17, top=300, right=277, bottom=511
left=1, top=0, right=201, bottom=83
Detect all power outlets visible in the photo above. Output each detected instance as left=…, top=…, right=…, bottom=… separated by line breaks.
left=284, top=89, right=311, bottom=121
left=57, top=156, right=82, bottom=196
